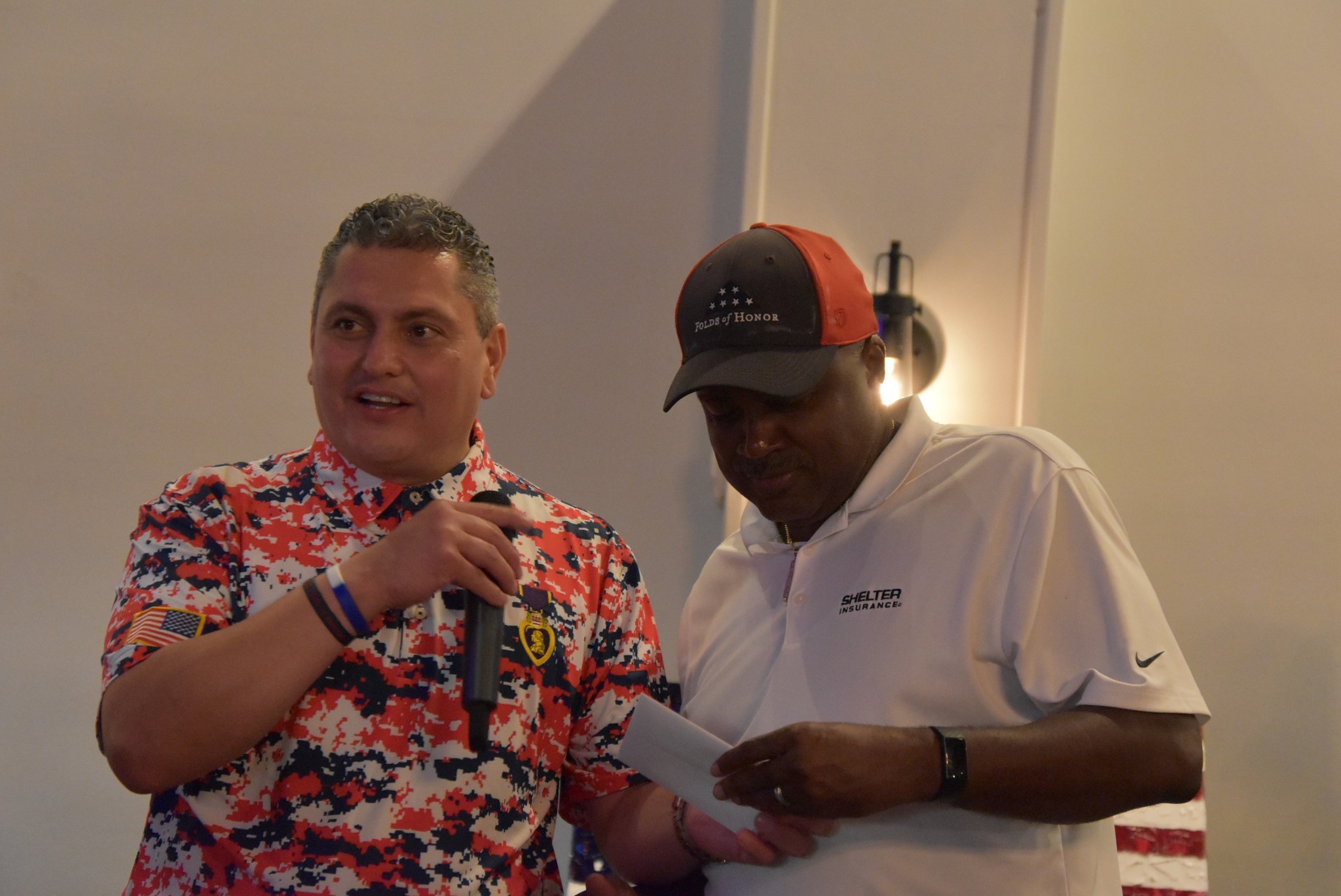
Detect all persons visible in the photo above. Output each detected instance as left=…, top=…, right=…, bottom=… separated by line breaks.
left=97, top=193, right=833, bottom=896
left=663, top=223, right=1211, bottom=896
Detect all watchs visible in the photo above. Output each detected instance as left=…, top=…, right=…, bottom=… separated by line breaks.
left=673, top=796, right=727, bottom=867
left=929, top=725, right=968, bottom=802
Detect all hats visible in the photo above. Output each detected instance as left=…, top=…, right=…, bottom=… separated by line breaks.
left=662, top=222, right=879, bottom=412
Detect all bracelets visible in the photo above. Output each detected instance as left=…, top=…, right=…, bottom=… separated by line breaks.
left=325, top=564, right=369, bottom=635
left=302, top=578, right=354, bottom=645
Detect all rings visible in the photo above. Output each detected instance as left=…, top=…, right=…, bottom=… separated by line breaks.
left=773, top=786, right=792, bottom=807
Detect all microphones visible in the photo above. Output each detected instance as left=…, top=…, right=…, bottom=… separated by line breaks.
left=465, top=489, right=516, bottom=754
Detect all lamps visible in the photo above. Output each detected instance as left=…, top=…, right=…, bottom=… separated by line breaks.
left=871, top=240, right=944, bottom=407
